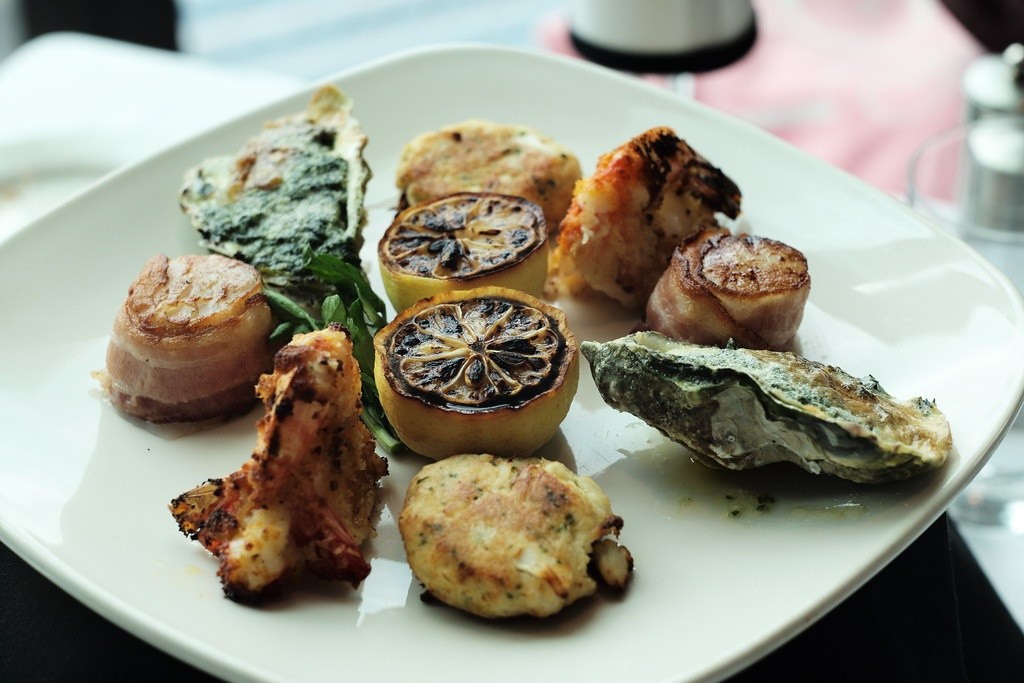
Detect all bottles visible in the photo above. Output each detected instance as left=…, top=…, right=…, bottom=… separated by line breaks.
left=944, top=42, right=1023, bottom=528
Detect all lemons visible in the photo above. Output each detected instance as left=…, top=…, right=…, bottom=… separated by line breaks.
left=372, top=286, right=579, bottom=462
left=378, top=193, right=554, bottom=315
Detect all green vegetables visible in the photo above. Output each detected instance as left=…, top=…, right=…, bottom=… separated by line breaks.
left=258, top=249, right=406, bottom=454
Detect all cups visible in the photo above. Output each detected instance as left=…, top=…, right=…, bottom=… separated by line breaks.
left=566, top=1, right=758, bottom=78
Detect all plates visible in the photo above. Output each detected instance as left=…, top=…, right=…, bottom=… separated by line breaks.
left=1, top=42, right=1021, bottom=683
